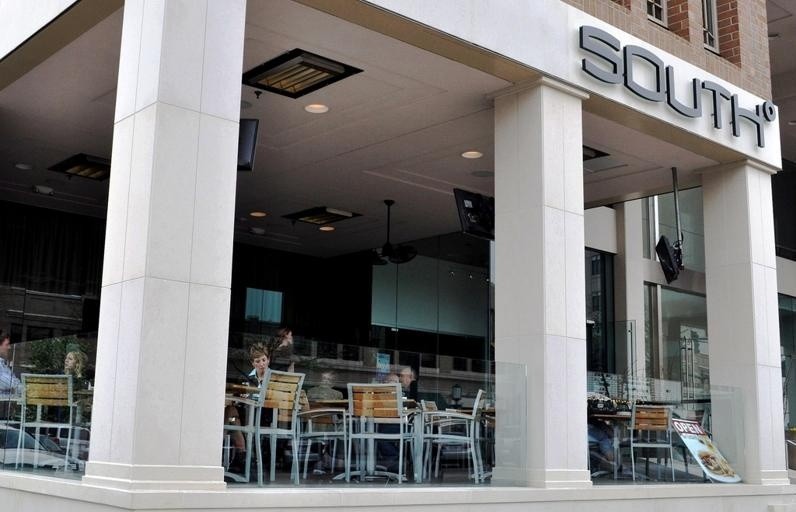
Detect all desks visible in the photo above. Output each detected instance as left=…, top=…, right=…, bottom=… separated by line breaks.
left=590, top=409, right=712, bottom=480
left=2, top=385, right=91, bottom=465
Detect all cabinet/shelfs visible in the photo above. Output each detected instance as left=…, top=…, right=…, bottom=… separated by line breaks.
left=369, top=256, right=487, bottom=339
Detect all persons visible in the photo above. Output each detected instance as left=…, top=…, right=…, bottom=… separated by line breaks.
left=225, top=343, right=275, bottom=474
left=379, top=366, right=419, bottom=471
left=306, top=369, right=342, bottom=475
left=49, top=350, right=88, bottom=435
left=590, top=419, right=625, bottom=478
left=268, top=327, right=296, bottom=372
left=0, top=334, right=21, bottom=392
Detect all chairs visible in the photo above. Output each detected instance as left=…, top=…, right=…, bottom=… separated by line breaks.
left=610, top=400, right=674, bottom=482
left=222, top=368, right=489, bottom=484
left=16, top=372, right=76, bottom=474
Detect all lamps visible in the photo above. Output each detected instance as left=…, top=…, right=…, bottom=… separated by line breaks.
left=654, top=162, right=688, bottom=283
left=378, top=198, right=419, bottom=266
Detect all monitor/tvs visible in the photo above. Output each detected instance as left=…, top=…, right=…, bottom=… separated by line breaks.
left=237, top=118, right=259, bottom=171
left=654, top=235, right=679, bottom=284
left=452, top=186, right=494, bottom=240
